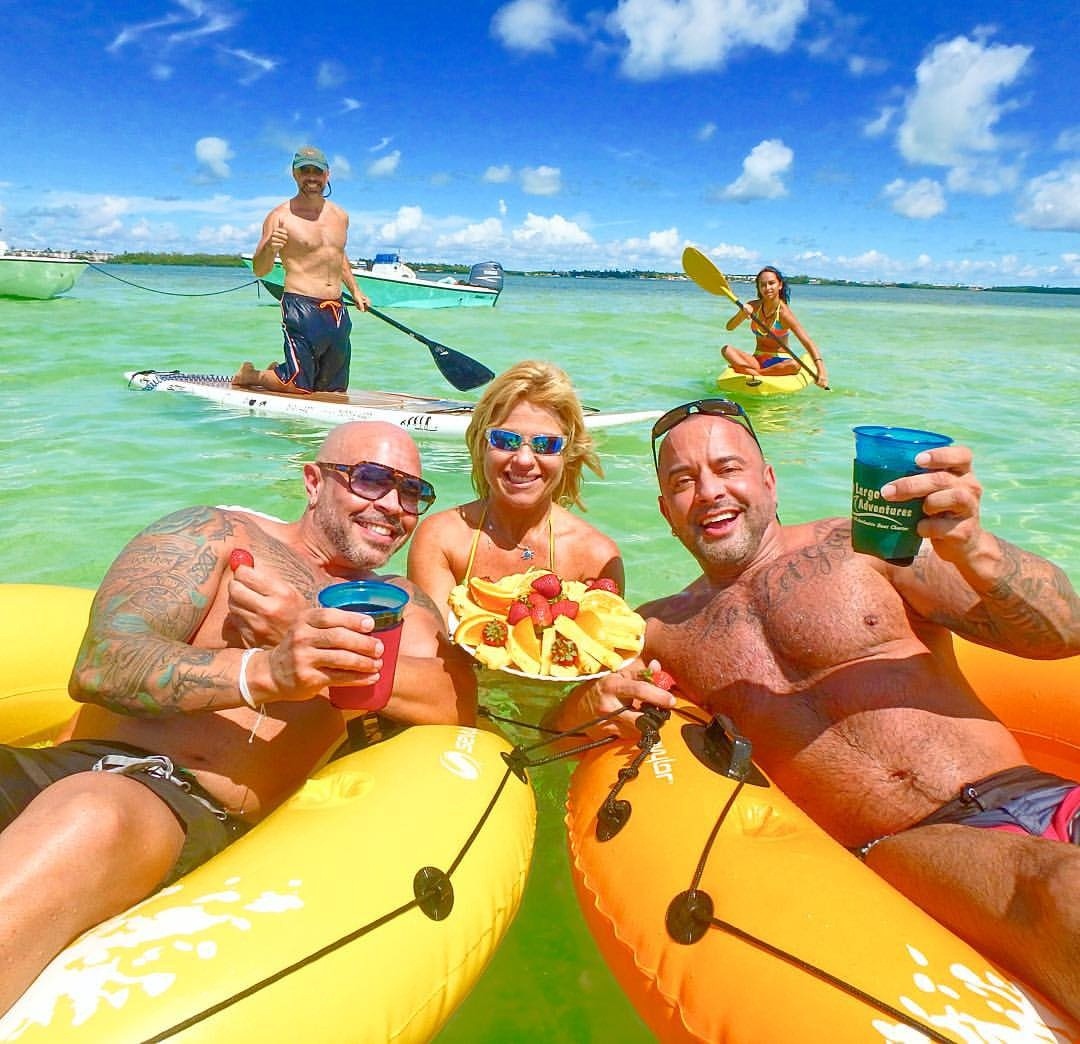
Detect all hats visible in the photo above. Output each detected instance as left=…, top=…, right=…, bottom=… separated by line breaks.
left=292, top=153, right=328, bottom=171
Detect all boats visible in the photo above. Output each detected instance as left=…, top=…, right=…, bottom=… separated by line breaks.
left=239, top=248, right=506, bottom=309
left=0, top=241, right=91, bottom=300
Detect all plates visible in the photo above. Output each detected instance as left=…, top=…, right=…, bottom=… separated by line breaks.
left=447, top=609, right=645, bottom=682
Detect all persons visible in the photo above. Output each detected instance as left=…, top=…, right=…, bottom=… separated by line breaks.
left=540, top=397, right=1080, bottom=1022
left=1, top=423, right=480, bottom=1021
left=231, top=146, right=370, bottom=394
left=721, top=266, right=828, bottom=388
left=406, top=361, right=625, bottom=633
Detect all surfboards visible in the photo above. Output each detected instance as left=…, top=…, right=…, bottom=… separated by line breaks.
left=122, top=368, right=668, bottom=442
left=716, top=352, right=817, bottom=397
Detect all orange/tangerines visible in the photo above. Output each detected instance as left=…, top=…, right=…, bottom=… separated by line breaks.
left=447, top=567, right=647, bottom=677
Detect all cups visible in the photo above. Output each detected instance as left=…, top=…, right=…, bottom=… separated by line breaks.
left=851, top=426, right=954, bottom=474
left=317, top=580, right=410, bottom=631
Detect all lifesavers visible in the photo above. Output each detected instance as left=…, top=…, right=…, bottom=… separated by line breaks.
left=1, top=581, right=538, bottom=1041
left=566, top=631, right=1080, bottom=1044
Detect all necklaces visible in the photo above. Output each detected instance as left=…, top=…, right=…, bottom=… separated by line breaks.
left=484, top=511, right=549, bottom=560
left=761, top=303, right=778, bottom=325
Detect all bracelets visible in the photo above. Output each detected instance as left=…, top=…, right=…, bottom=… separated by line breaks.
left=238, top=648, right=269, bottom=743
left=814, top=359, right=823, bottom=362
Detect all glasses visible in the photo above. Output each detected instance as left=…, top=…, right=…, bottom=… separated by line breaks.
left=651, top=398, right=763, bottom=497
left=297, top=167, right=323, bottom=176
left=315, top=460, right=436, bottom=516
left=485, top=427, right=567, bottom=456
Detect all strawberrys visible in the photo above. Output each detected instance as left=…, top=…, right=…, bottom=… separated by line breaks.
left=567, top=601, right=579, bottom=620
left=550, top=597, right=570, bottom=620
left=530, top=600, right=554, bottom=640
left=585, top=577, right=619, bottom=595
left=507, top=594, right=530, bottom=625
left=531, top=574, right=563, bottom=599
left=230, top=548, right=255, bottom=571
left=482, top=620, right=507, bottom=646
left=552, top=635, right=577, bottom=666
left=637, top=669, right=677, bottom=690
left=527, top=591, right=549, bottom=604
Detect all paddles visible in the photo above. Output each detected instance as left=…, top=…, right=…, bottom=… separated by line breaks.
left=341, top=290, right=493, bottom=392
left=682, top=245, right=832, bottom=393
